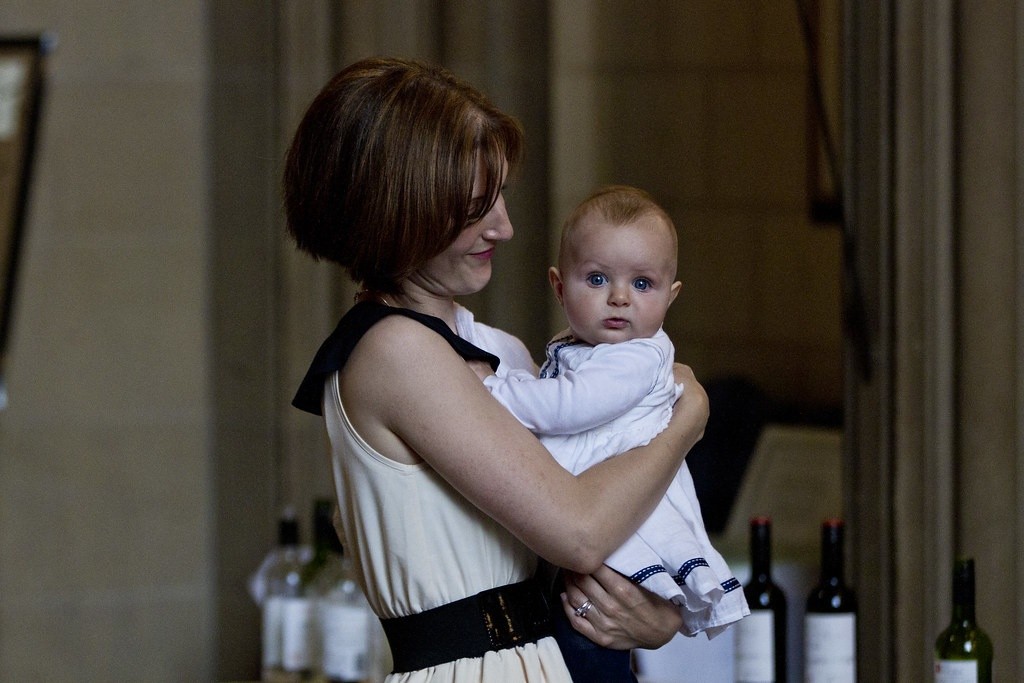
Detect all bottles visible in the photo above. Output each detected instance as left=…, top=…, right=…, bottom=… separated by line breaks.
left=733, top=516, right=789, bottom=683
left=931, top=548, right=994, bottom=683
left=258, top=506, right=305, bottom=683
left=295, top=498, right=345, bottom=683
left=800, top=519, right=860, bottom=683
left=315, top=554, right=383, bottom=683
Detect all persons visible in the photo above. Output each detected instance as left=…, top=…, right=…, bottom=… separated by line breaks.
left=282, top=54, right=750, bottom=683
left=465, top=184, right=751, bottom=683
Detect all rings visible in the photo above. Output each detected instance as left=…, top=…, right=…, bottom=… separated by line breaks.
left=575, top=600, right=594, bottom=618
